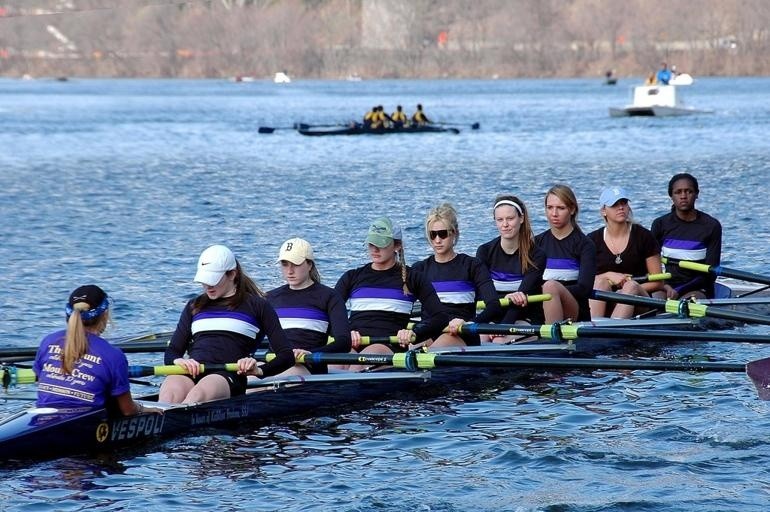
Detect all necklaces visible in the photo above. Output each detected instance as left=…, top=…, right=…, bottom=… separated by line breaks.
left=607, top=229, right=630, bottom=264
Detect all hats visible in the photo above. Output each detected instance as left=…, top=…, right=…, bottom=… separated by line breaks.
left=363, top=216, right=402, bottom=249
left=276, top=237, right=312, bottom=266
left=65, top=285, right=109, bottom=319
left=193, top=245, right=237, bottom=287
left=600, top=185, right=631, bottom=209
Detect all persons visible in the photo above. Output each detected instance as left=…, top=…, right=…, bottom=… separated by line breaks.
left=476, top=197, right=547, bottom=346
left=0, top=363, right=17, bottom=392
left=245, top=238, right=351, bottom=393
left=651, top=174, right=722, bottom=299
left=157, top=244, right=296, bottom=412
left=328, top=218, right=449, bottom=371
left=531, top=185, right=594, bottom=323
left=584, top=186, right=664, bottom=321
left=645, top=63, right=677, bottom=85
left=29, top=285, right=163, bottom=426
left=364, top=104, right=432, bottom=125
left=408, top=204, right=501, bottom=348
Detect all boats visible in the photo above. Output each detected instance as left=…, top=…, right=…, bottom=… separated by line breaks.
left=0, top=285, right=770, bottom=467
left=608, top=73, right=714, bottom=116
left=601, top=78, right=618, bottom=85
left=298, top=125, right=458, bottom=136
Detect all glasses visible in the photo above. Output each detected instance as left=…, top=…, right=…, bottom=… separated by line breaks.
left=429, top=229, right=449, bottom=240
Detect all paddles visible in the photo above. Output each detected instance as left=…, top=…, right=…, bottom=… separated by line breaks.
left=258, top=126, right=350, bottom=134
left=432, top=121, right=480, bottom=129
left=251, top=349, right=769, bottom=400
left=293, top=122, right=348, bottom=130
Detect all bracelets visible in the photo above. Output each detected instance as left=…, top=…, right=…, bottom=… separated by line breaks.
left=137, top=402, right=144, bottom=414
